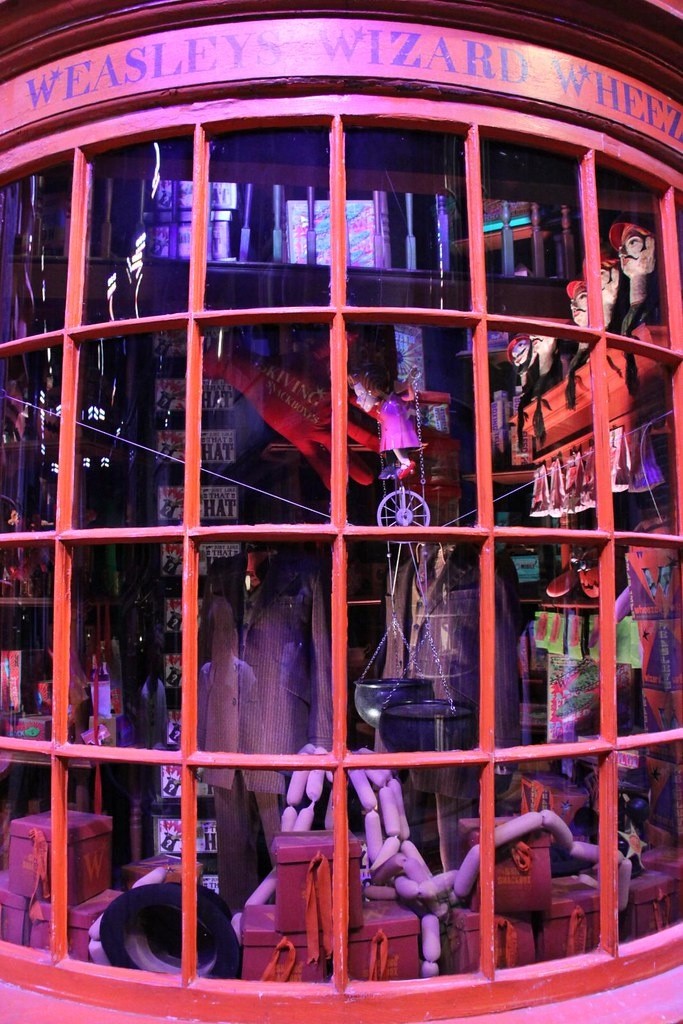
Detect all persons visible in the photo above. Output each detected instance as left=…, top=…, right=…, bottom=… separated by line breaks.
left=508, top=218, right=664, bottom=597
left=201, top=547, right=329, bottom=916
left=139, top=651, right=168, bottom=862
left=376, top=543, right=512, bottom=869
left=347, top=365, right=419, bottom=479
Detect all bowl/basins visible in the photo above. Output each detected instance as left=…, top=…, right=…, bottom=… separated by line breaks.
left=355, top=677, right=434, bottom=729
left=379, top=698, right=477, bottom=752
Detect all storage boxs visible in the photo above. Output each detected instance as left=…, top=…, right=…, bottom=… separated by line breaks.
left=141, top=175, right=396, bottom=273
left=239, top=515, right=683, bottom=985
left=0, top=648, right=203, bottom=964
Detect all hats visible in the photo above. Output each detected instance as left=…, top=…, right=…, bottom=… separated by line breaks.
left=583, top=247, right=621, bottom=280
left=609, top=214, right=657, bottom=251
left=567, top=272, right=586, bottom=299
left=99, top=882, right=241, bottom=980
left=507, top=333, right=529, bottom=364
